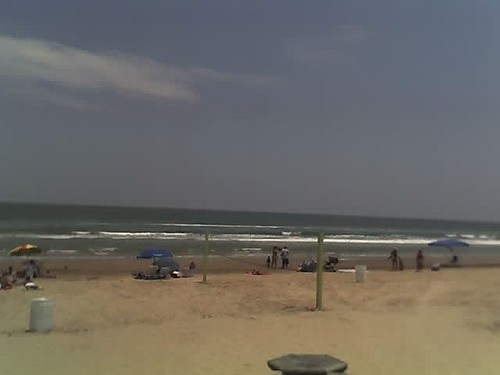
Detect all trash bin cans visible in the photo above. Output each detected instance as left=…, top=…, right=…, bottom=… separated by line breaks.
left=355, top=265, right=367, bottom=282
left=30, top=298, right=54, bottom=332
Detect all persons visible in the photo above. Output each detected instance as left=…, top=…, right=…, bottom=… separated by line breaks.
left=267, top=255, right=270, bottom=267
left=188, top=261, right=196, bottom=273
left=155, top=264, right=161, bottom=275
left=271, top=246, right=278, bottom=268
left=28, top=260, right=39, bottom=278
left=280, top=247, right=290, bottom=269
left=415, top=250, right=423, bottom=272
left=387, top=249, right=399, bottom=271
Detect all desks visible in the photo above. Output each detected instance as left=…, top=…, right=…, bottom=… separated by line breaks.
left=267, top=353, right=347, bottom=375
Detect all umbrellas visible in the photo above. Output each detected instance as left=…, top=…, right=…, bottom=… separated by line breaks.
left=428, top=238, right=470, bottom=263
left=135, top=249, right=175, bottom=265
left=9, top=244, right=45, bottom=262
